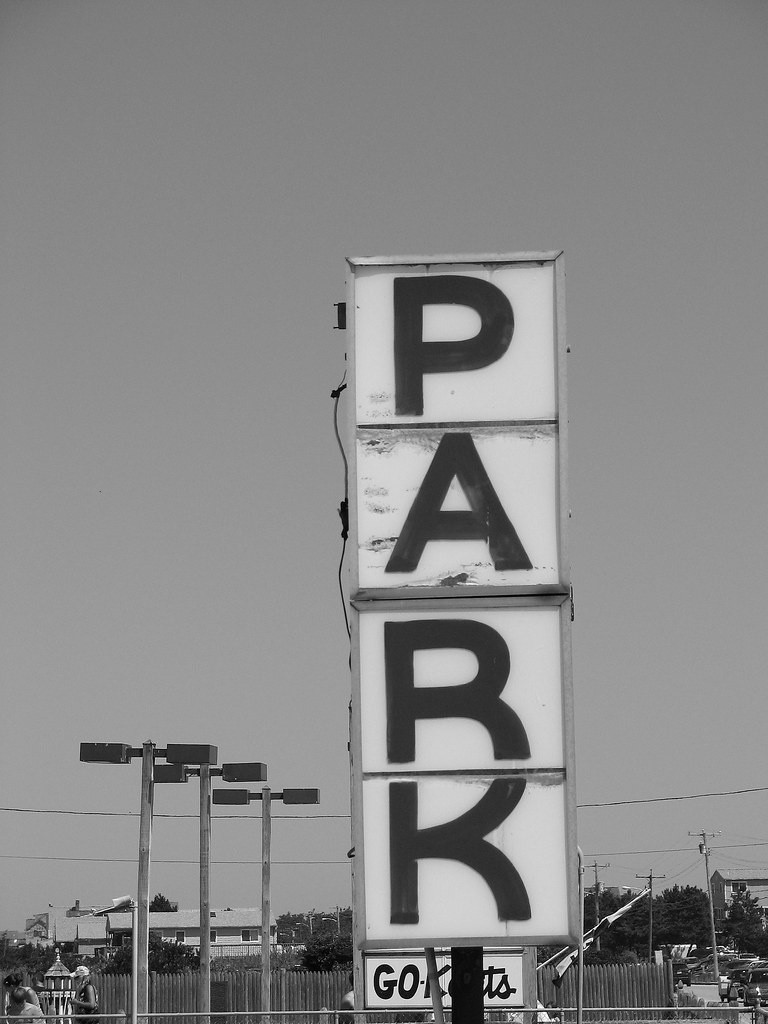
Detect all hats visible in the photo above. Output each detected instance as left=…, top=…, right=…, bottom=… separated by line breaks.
left=70, top=965, right=89, bottom=978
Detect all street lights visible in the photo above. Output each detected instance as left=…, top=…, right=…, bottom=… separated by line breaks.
left=322, top=905, right=340, bottom=934
left=213, top=788, right=321, bottom=1024
left=154, top=762, right=268, bottom=1024
left=296, top=912, right=316, bottom=935
left=79, top=738, right=218, bottom=1024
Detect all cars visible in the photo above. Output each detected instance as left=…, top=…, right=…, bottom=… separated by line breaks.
left=740, top=968, right=768, bottom=1007
left=660, top=944, right=768, bottom=1003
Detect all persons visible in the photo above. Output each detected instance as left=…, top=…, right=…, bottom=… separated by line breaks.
left=339, top=970, right=561, bottom=1024
left=2, top=964, right=51, bottom=1024
left=68, top=966, right=100, bottom=1024
